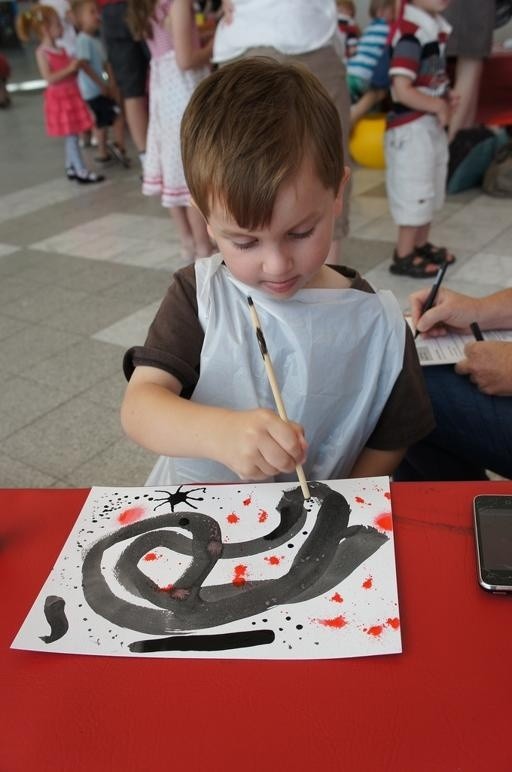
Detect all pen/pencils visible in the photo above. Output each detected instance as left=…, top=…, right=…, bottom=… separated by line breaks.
left=414, top=260, right=449, bottom=341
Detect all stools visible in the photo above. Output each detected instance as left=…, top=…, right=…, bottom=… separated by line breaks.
left=348, top=112, right=387, bottom=170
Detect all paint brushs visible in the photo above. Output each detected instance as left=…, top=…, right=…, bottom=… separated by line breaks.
left=247, top=296, right=312, bottom=512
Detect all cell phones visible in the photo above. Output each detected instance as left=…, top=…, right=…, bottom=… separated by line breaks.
left=473, top=494, right=512, bottom=595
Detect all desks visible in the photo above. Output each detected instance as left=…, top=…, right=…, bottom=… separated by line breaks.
left=0, top=480, right=512, bottom=772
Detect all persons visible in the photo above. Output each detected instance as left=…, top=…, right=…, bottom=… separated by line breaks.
left=119, top=57, right=439, bottom=487
left=386, top=282, right=512, bottom=483
left=1, top=1, right=511, bottom=278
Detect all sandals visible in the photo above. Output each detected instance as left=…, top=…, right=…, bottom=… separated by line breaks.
left=390, top=248, right=436, bottom=277
left=66, top=138, right=147, bottom=186
left=415, top=242, right=455, bottom=265
